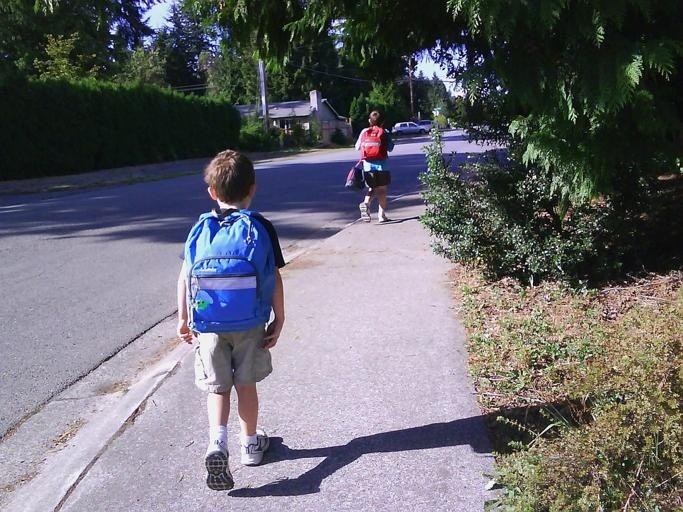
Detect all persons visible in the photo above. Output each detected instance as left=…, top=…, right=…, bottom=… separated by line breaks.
left=355, top=110, right=395, bottom=223
left=177, top=148, right=284, bottom=491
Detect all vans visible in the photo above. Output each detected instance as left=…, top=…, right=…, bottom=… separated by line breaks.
left=416, top=120, right=433, bottom=131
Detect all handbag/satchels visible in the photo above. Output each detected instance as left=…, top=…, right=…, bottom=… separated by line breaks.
left=344, top=160, right=366, bottom=194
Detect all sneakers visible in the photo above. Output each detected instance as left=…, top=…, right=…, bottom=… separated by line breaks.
left=205, top=438, right=235, bottom=489
left=379, top=214, right=391, bottom=223
left=359, top=202, right=372, bottom=223
left=240, top=428, right=270, bottom=467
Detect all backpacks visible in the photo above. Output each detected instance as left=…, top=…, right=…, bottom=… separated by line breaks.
left=185, top=210, right=275, bottom=332
left=358, top=127, right=387, bottom=161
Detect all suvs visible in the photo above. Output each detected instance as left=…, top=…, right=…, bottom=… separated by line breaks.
left=390, top=122, right=429, bottom=135
